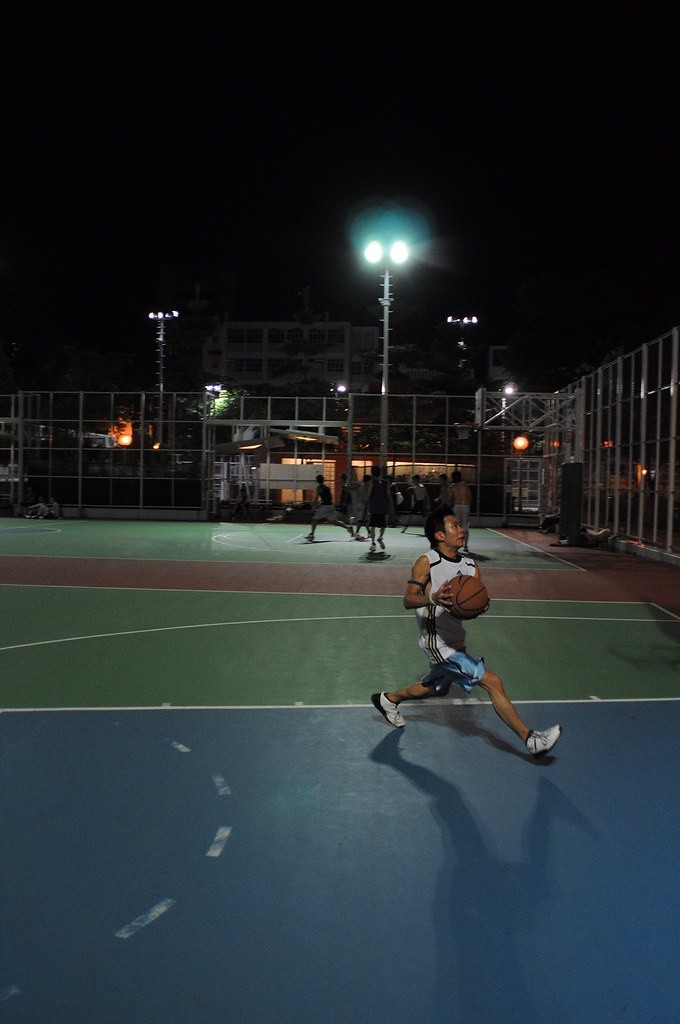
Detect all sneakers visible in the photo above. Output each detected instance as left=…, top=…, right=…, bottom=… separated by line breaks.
left=525, top=724, right=562, bottom=760
left=371, top=692, right=406, bottom=728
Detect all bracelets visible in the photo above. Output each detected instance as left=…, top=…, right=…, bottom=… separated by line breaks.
left=428, top=593, right=436, bottom=606
left=439, top=498, right=440, bottom=500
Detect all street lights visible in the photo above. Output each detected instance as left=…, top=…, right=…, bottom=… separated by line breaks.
left=512, top=435, right=528, bottom=514
left=148, top=311, right=179, bottom=446
left=446, top=315, right=480, bottom=468
left=362, top=238, right=409, bottom=483
left=117, top=434, right=132, bottom=471
left=498, top=385, right=514, bottom=454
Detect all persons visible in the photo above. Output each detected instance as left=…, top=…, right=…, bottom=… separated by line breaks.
left=355, top=475, right=373, bottom=539
left=539, top=498, right=561, bottom=534
left=368, top=466, right=392, bottom=551
left=24, top=486, right=59, bottom=518
left=447, top=471, right=471, bottom=551
left=402, top=475, right=430, bottom=533
left=231, top=489, right=247, bottom=521
left=340, top=473, right=351, bottom=523
left=305, top=475, right=354, bottom=540
left=373, top=509, right=562, bottom=761
left=433, top=474, right=450, bottom=508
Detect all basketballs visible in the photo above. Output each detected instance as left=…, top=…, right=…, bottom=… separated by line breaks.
left=443, top=574, right=489, bottom=621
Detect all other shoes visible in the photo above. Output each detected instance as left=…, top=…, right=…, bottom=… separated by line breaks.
left=378, top=537, right=386, bottom=549
left=354, top=533, right=360, bottom=537
left=304, top=533, right=314, bottom=540
left=370, top=545, right=376, bottom=551
left=367, top=533, right=372, bottom=538
left=347, top=526, right=354, bottom=537
